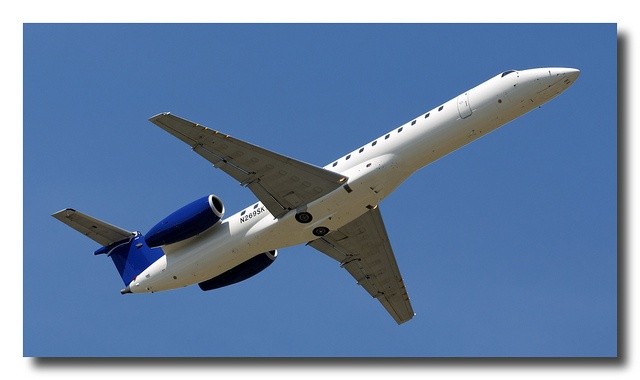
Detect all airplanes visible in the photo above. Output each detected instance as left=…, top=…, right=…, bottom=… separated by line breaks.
left=49, top=65, right=581, bottom=326
